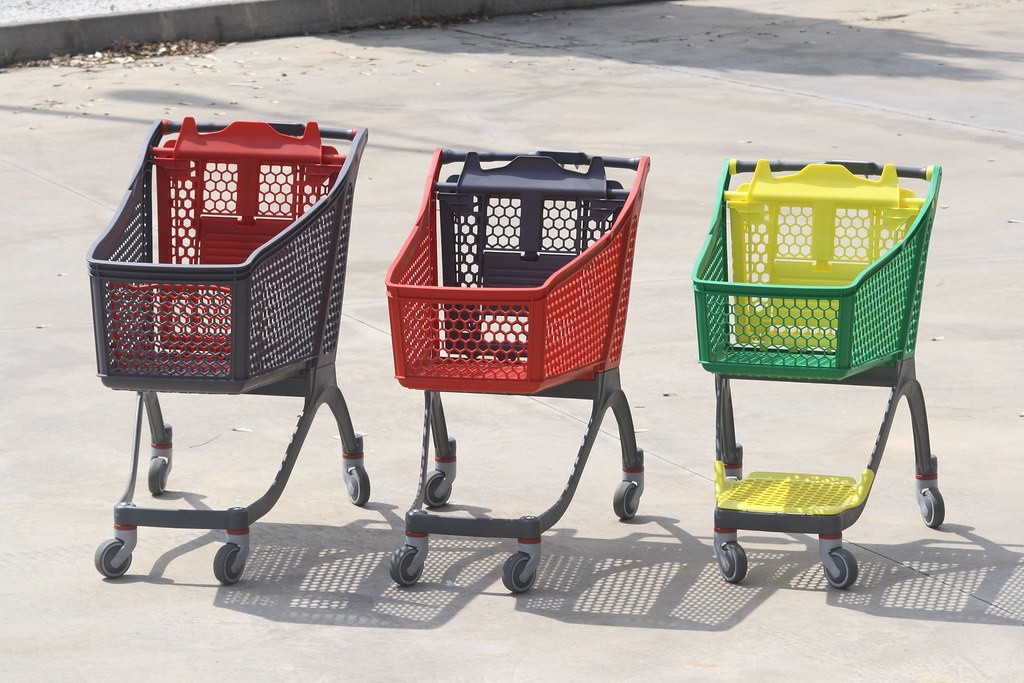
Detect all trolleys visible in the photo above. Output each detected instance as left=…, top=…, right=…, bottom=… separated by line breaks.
left=382, top=144, right=655, bottom=595
left=688, top=154, right=947, bottom=592
left=85, top=114, right=374, bottom=588
